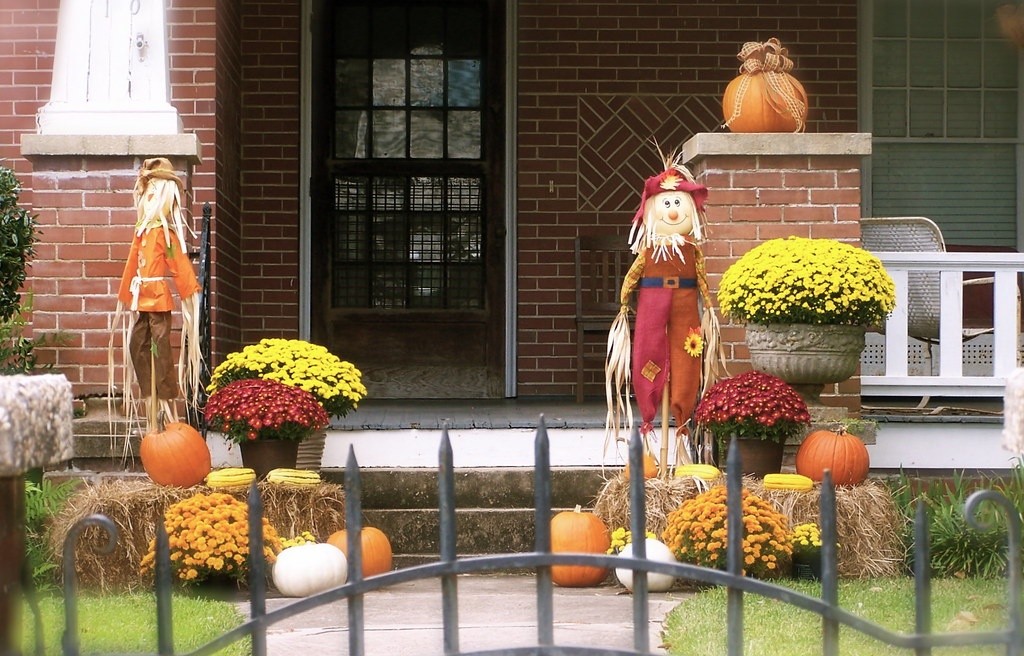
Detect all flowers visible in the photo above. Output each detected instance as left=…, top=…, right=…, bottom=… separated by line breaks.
left=203, top=338, right=368, bottom=421
left=716, top=235, right=896, bottom=329
left=206, top=379, right=330, bottom=442
left=661, top=484, right=793, bottom=579
left=137, top=493, right=282, bottom=582
left=281, top=530, right=315, bottom=549
left=606, top=526, right=657, bottom=554
left=789, top=522, right=840, bottom=549
left=694, top=372, right=814, bottom=442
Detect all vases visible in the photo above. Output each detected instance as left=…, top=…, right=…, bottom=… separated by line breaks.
left=238, top=437, right=299, bottom=478
left=720, top=436, right=783, bottom=480
left=743, top=318, right=864, bottom=401
left=790, top=548, right=824, bottom=579
left=295, top=421, right=327, bottom=474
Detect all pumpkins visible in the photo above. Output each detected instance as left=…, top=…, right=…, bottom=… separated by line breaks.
left=616, top=539, right=676, bottom=591
left=675, top=464, right=721, bottom=482
left=140, top=410, right=211, bottom=487
left=764, top=474, right=813, bottom=491
left=267, top=468, right=321, bottom=485
left=796, top=424, right=869, bottom=487
left=272, top=540, right=348, bottom=597
left=550, top=505, right=609, bottom=586
left=203, top=469, right=255, bottom=488
left=626, top=454, right=657, bottom=478
left=723, top=72, right=809, bottom=133
left=327, top=527, right=392, bottom=578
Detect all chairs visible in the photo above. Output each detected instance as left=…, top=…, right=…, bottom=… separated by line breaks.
left=859, top=217, right=994, bottom=375
